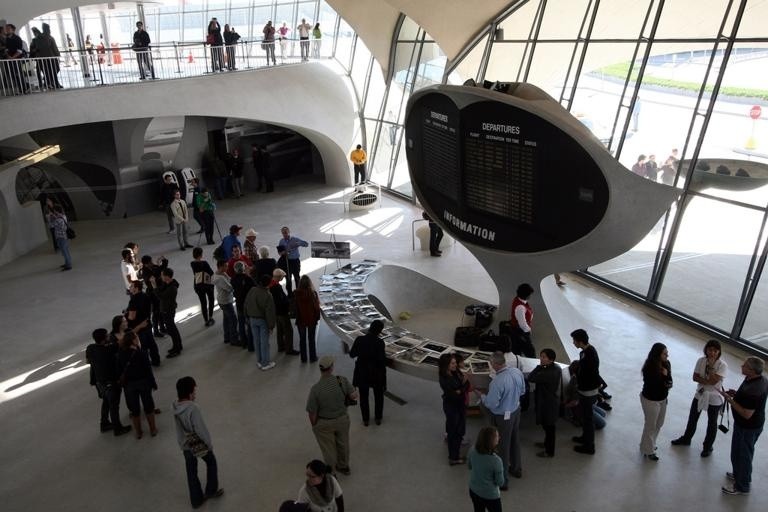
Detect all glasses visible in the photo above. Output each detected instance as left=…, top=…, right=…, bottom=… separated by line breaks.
left=306, top=472, right=316, bottom=479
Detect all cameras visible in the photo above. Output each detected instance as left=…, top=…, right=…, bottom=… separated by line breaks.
left=212, top=17, right=217, bottom=20
left=266, top=24, right=271, bottom=26
left=344, top=395, right=357, bottom=407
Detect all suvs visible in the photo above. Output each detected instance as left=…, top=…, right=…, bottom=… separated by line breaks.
left=394, top=64, right=464, bottom=97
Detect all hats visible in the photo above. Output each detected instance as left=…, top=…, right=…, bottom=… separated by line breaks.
left=246, top=229, right=259, bottom=236
left=319, top=356, right=333, bottom=369
left=273, top=268, right=286, bottom=276
left=230, top=224, right=242, bottom=234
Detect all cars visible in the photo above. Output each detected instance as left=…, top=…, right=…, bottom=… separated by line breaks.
left=574, top=113, right=615, bottom=156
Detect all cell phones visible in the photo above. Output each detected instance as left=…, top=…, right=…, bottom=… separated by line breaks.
left=232, top=28, right=234, bottom=30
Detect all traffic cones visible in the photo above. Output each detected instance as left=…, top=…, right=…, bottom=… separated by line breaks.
left=188, top=49, right=196, bottom=64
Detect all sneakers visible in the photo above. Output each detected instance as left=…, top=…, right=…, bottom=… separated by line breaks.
left=205, top=489, right=224, bottom=498
left=727, top=471, right=734, bottom=482
left=205, top=318, right=214, bottom=326
left=449, top=458, right=464, bottom=464
left=193, top=495, right=207, bottom=509
left=556, top=282, right=566, bottom=286
left=645, top=437, right=713, bottom=461
left=336, top=464, right=349, bottom=475
left=224, top=337, right=317, bottom=370
left=536, top=436, right=594, bottom=457
left=160, top=327, right=167, bottom=332
left=154, top=331, right=164, bottom=337
left=168, top=346, right=183, bottom=352
left=167, top=351, right=179, bottom=358
left=437, top=250, right=442, bottom=253
left=510, top=468, right=521, bottom=479
left=431, top=252, right=440, bottom=256
left=722, top=485, right=748, bottom=495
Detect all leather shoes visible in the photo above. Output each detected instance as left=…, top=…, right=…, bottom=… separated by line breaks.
left=101, top=408, right=161, bottom=440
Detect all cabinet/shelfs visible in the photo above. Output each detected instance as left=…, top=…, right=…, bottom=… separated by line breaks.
left=317, top=256, right=579, bottom=428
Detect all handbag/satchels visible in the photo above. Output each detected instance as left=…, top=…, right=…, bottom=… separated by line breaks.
left=186, top=432, right=208, bottom=456
left=66, top=228, right=75, bottom=240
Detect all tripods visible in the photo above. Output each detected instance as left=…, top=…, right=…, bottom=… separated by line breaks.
left=323, top=228, right=341, bottom=275
left=196, top=203, right=222, bottom=246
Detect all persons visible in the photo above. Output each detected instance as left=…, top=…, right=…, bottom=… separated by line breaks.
left=640, top=343, right=673, bottom=461
left=511, top=283, right=537, bottom=359
left=671, top=340, right=728, bottom=457
left=0, top=22, right=105, bottom=94
left=173, top=377, right=224, bottom=509
left=295, top=460, right=345, bottom=510
left=349, top=321, right=387, bottom=426
left=86, top=243, right=183, bottom=440
left=206, top=17, right=240, bottom=71
left=43, top=196, right=73, bottom=271
left=467, top=426, right=504, bottom=510
left=209, top=143, right=274, bottom=200
left=191, top=225, right=320, bottom=370
left=717, top=356, right=766, bottom=495
left=156, top=175, right=216, bottom=251
left=350, top=145, right=367, bottom=186
left=306, top=356, right=358, bottom=475
left=439, top=329, right=612, bottom=491
left=132, top=21, right=156, bottom=80
left=631, top=148, right=677, bottom=186
left=262, top=19, right=321, bottom=66
left=422, top=210, right=444, bottom=257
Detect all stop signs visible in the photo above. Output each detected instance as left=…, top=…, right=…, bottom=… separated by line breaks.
left=750, top=106, right=761, bottom=119
